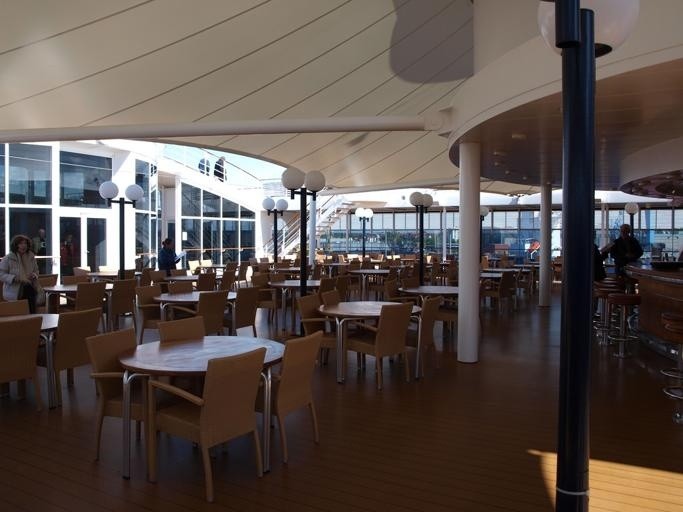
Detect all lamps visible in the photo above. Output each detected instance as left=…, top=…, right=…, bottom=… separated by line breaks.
left=539, top=0, right=640, bottom=511
left=354, top=208, right=374, bottom=257
left=409, top=193, right=433, bottom=281
left=479, top=204, right=489, bottom=263
left=94, top=179, right=145, bottom=270
left=262, top=197, right=288, bottom=260
left=279, top=168, right=326, bottom=296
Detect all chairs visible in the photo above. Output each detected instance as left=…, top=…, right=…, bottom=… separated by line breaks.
left=274, top=262, right=291, bottom=267
left=39, top=274, right=58, bottom=301
left=235, top=262, right=249, bottom=288
left=249, top=258, right=257, bottom=264
left=142, top=268, right=155, bottom=285
left=150, top=270, right=166, bottom=283
left=168, top=281, right=193, bottom=293
left=220, top=271, right=235, bottom=289
left=519, top=268, right=536, bottom=294
left=439, top=308, right=457, bottom=346
left=40, top=308, right=101, bottom=409
left=322, top=290, right=361, bottom=371
left=384, top=280, right=404, bottom=302
left=225, top=287, right=257, bottom=337
left=259, top=257, right=269, bottom=263
left=225, top=262, right=238, bottom=272
left=253, top=330, right=323, bottom=465
left=315, top=277, right=335, bottom=293
left=85, top=326, right=144, bottom=463
left=197, top=273, right=216, bottom=290
left=173, top=269, right=186, bottom=277
left=73, top=267, right=90, bottom=278
left=138, top=285, right=166, bottom=343
left=484, top=270, right=518, bottom=315
left=400, top=278, right=421, bottom=287
left=337, top=274, right=349, bottom=300
left=118, top=271, right=136, bottom=287
left=147, top=345, right=267, bottom=504
left=0, top=298, right=30, bottom=318
left=297, top=294, right=336, bottom=367
left=250, top=270, right=294, bottom=327
left=315, top=248, right=566, bottom=279
left=74, top=282, right=106, bottom=310
left=171, top=290, right=225, bottom=336
left=107, top=279, right=138, bottom=334
left=257, top=263, right=269, bottom=272
left=345, top=302, right=415, bottom=391
left=62, top=276, right=90, bottom=304
left=0, top=318, right=45, bottom=413
left=156, top=315, right=204, bottom=343
left=408, top=296, right=442, bottom=384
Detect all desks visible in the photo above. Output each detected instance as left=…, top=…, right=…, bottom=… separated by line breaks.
left=398, top=286, right=458, bottom=354
left=349, top=270, right=390, bottom=302
left=114, top=336, right=286, bottom=481
left=268, top=278, right=321, bottom=330
left=476, top=272, right=508, bottom=307
left=42, top=283, right=119, bottom=313
left=318, top=300, right=422, bottom=383
left=154, top=292, right=238, bottom=321
left=0, top=313, right=84, bottom=407
left=89, top=270, right=144, bottom=279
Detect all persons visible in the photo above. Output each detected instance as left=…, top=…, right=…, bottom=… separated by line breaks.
left=609, top=224, right=644, bottom=294
left=590, top=229, right=605, bottom=319
left=28, top=228, right=49, bottom=271
left=158, top=239, right=181, bottom=275
left=60, top=233, right=77, bottom=276
left=0, top=235, right=42, bottom=314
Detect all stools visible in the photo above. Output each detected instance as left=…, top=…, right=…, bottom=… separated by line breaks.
left=608, top=292, right=642, bottom=362
left=595, top=282, right=616, bottom=336
left=663, top=323, right=683, bottom=401
left=598, top=288, right=624, bottom=347
left=595, top=278, right=622, bottom=323
left=661, top=310, right=683, bottom=378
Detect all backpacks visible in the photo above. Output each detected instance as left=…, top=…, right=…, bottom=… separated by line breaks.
left=34, top=279, right=46, bottom=306
